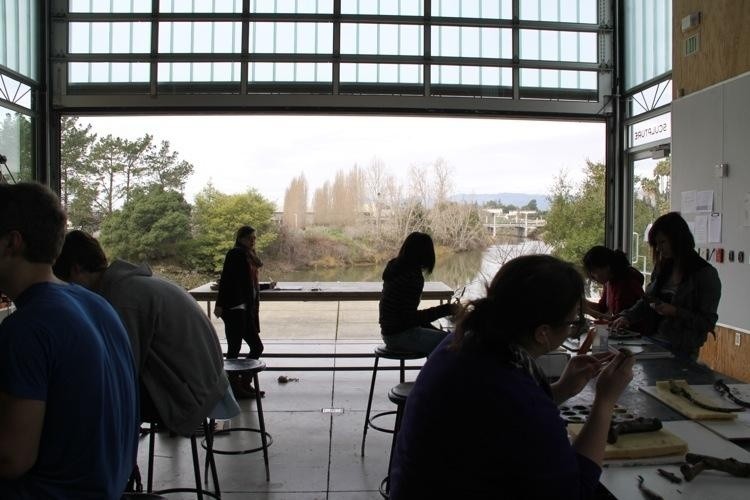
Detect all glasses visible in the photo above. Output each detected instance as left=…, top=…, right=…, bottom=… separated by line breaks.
left=565, top=293, right=587, bottom=325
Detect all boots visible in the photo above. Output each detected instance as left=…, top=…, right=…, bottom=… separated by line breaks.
left=230, top=379, right=265, bottom=398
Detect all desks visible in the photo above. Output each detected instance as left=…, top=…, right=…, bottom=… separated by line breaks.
left=187, top=280, right=455, bottom=339
left=543, top=316, right=750, bottom=500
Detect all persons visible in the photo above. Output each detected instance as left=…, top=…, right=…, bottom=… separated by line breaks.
left=52, top=229, right=241, bottom=436
left=388, top=254, right=637, bottom=499
left=0, top=181, right=142, bottom=499
left=602, top=211, right=722, bottom=364
left=377, top=231, right=460, bottom=359
left=580, top=244, right=647, bottom=332
left=213, top=226, right=266, bottom=403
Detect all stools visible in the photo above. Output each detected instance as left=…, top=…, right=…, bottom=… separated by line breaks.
left=361, top=346, right=425, bottom=458
left=379, top=382, right=418, bottom=500
left=124, top=359, right=272, bottom=500
